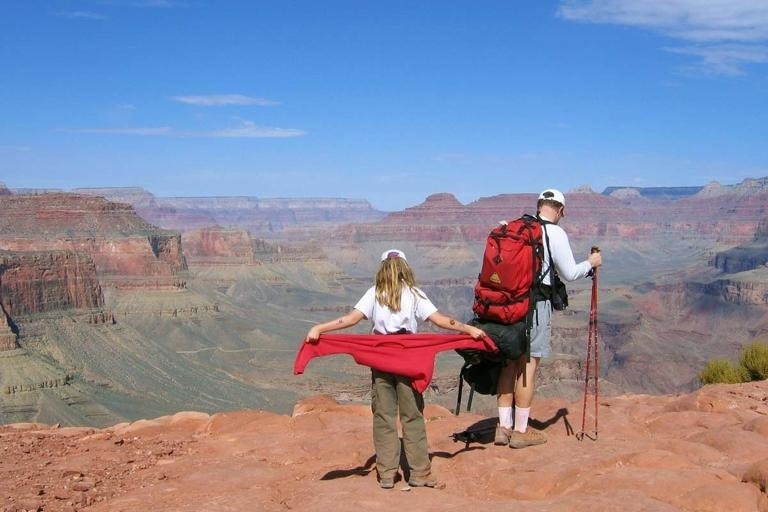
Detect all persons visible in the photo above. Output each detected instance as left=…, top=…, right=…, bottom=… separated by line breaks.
left=493, top=188, right=603, bottom=449
left=303, top=249, right=488, bottom=490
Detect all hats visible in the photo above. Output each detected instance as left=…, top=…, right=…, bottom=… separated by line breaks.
left=537, top=189, right=565, bottom=207
left=380, top=249, right=407, bottom=263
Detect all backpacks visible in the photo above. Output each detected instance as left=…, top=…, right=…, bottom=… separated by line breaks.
left=466, top=317, right=525, bottom=360
left=472, top=213, right=556, bottom=326
left=462, top=357, right=503, bottom=397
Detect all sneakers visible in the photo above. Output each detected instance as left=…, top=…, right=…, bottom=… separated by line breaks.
left=408, top=473, right=446, bottom=489
left=494, top=422, right=512, bottom=445
left=509, top=426, right=547, bottom=449
left=379, top=478, right=394, bottom=488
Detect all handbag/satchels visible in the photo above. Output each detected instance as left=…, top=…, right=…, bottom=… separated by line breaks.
left=550, top=268, right=569, bottom=312
